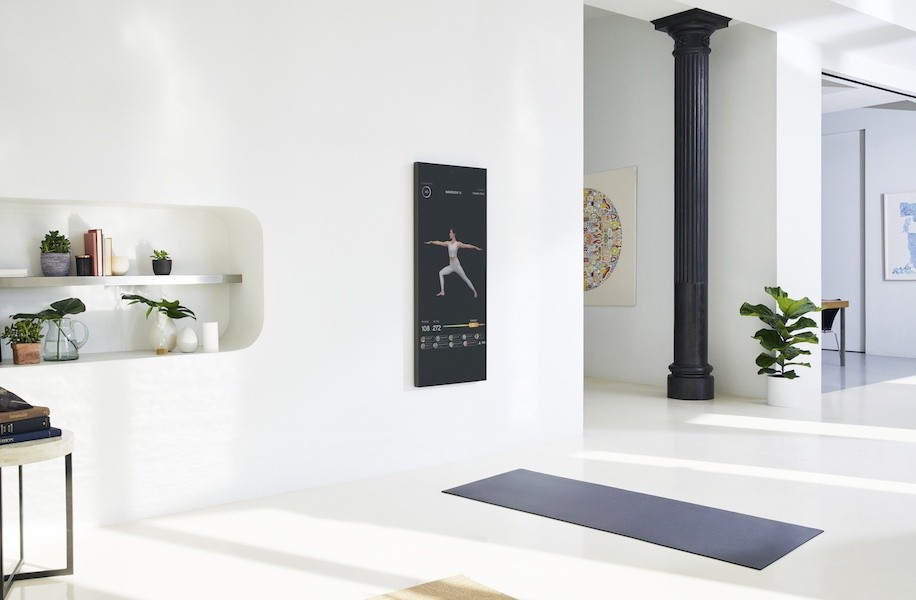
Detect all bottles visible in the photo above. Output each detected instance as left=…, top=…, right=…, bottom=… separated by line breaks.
left=75, top=255, right=91, bottom=276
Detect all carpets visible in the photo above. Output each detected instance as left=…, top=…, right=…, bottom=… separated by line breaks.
left=441, top=466, right=826, bottom=569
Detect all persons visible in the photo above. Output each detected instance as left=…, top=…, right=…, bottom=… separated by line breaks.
left=424, top=224, right=483, bottom=298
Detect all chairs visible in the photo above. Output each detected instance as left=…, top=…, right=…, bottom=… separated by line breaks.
left=821, top=298, right=849, bottom=366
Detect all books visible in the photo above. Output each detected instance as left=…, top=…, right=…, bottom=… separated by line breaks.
left=0, top=386, right=61, bottom=445
left=84, top=229, right=112, bottom=277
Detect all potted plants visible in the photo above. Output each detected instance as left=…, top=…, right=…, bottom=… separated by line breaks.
left=151, top=248, right=172, bottom=275
left=739, top=286, right=826, bottom=408
left=1, top=319, right=45, bottom=365
left=120, top=291, right=198, bottom=351
left=38, top=230, right=74, bottom=277
left=11, top=296, right=89, bottom=363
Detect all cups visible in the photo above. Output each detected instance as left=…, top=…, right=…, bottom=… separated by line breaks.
left=202, top=322, right=220, bottom=353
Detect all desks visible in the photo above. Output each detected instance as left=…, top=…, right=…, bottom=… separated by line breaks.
left=0, top=427, right=76, bottom=600
